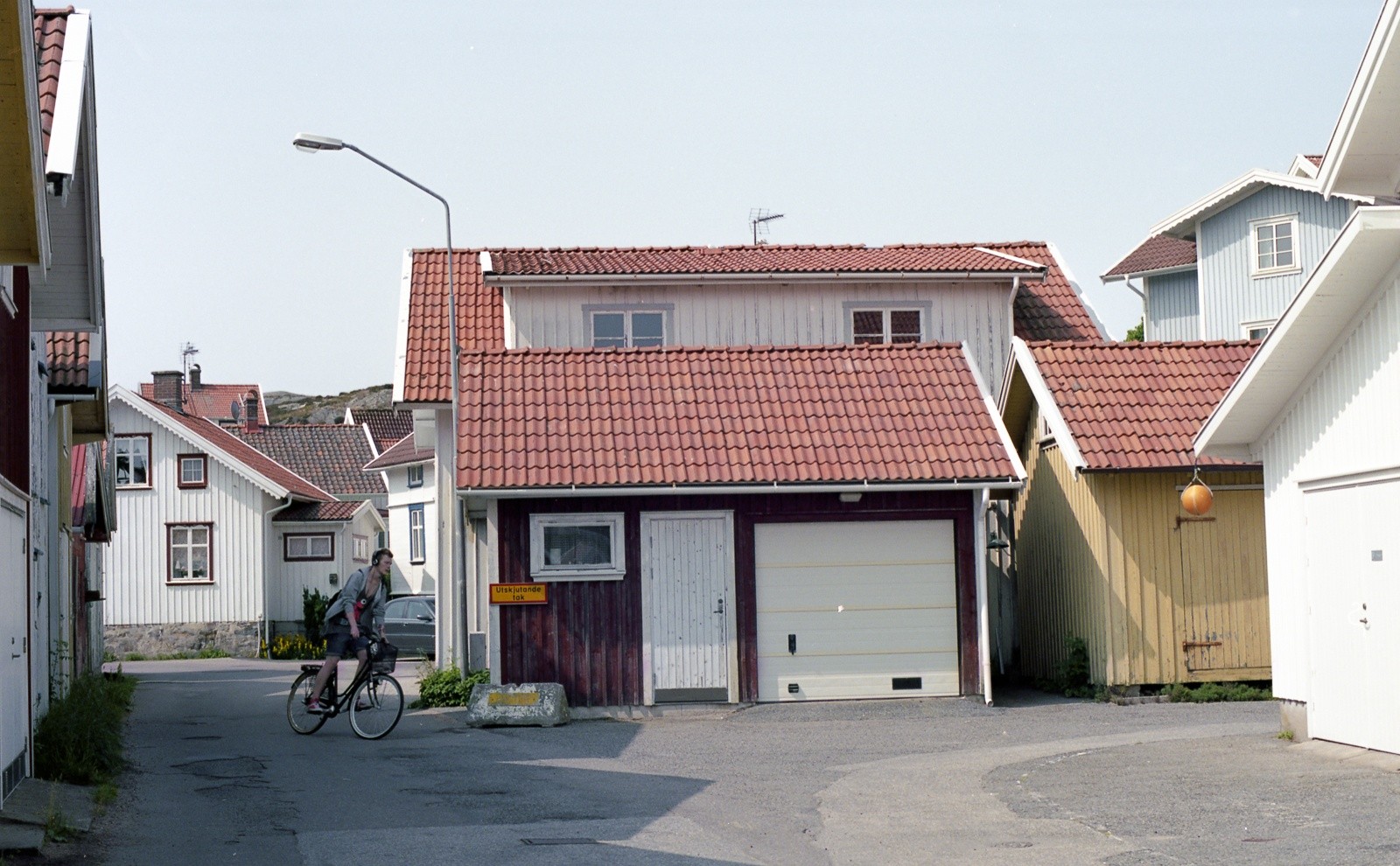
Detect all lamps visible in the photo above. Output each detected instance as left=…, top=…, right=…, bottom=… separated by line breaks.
left=838, top=492, right=862, bottom=502
left=987, top=539, right=1013, bottom=557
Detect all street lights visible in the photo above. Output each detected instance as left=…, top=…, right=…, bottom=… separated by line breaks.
left=293, top=134, right=467, bottom=681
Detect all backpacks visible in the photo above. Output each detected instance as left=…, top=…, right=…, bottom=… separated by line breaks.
left=327, top=568, right=383, bottom=627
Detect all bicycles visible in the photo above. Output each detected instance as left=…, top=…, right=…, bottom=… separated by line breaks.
left=286, top=632, right=405, bottom=741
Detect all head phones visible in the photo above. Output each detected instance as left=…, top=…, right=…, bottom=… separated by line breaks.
left=372, top=549, right=383, bottom=566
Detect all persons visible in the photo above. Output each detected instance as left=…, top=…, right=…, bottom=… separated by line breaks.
left=305, top=548, right=392, bottom=714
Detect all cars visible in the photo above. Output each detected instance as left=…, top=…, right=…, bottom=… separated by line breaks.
left=370, top=595, right=435, bottom=657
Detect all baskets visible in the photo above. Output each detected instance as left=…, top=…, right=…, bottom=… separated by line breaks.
left=369, top=641, right=398, bottom=674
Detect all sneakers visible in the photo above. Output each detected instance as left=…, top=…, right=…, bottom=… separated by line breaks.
left=347, top=695, right=372, bottom=711
left=307, top=698, right=323, bottom=714
